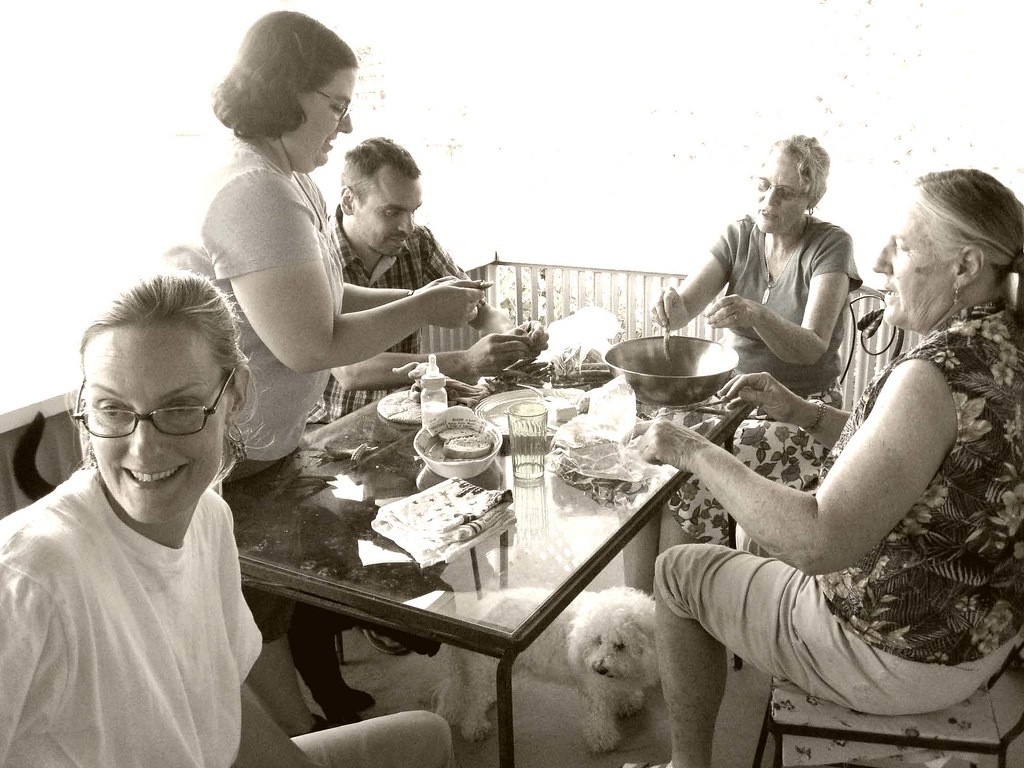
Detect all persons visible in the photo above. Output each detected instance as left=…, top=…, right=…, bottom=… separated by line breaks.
left=167, top=11, right=549, bottom=738
left=0, top=271, right=457, bottom=768
left=620, top=169, right=1024, bottom=768
left=621, top=135, right=863, bottom=601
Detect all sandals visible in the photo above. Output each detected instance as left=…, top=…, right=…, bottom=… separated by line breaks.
left=620, top=763, right=667, bottom=768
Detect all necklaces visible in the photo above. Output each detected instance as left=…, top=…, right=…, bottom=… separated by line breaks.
left=761, top=235, right=796, bottom=305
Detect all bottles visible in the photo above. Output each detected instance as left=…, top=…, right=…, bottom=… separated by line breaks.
left=420, top=354, right=448, bottom=429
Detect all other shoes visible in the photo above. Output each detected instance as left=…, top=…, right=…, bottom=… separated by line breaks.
left=362, top=628, right=408, bottom=655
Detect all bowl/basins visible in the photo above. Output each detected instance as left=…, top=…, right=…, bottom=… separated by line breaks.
left=414, top=406, right=504, bottom=480
left=602, top=335, right=740, bottom=406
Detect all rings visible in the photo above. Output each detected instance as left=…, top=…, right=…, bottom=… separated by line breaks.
left=732, top=313, right=737, bottom=322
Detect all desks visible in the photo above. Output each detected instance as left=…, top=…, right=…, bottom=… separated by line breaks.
left=212, top=347, right=758, bottom=768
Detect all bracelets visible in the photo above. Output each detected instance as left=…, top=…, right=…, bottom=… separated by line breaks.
left=407, top=289, right=415, bottom=296
left=799, top=400, right=826, bottom=433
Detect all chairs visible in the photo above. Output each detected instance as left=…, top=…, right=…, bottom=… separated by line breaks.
left=832, top=284, right=905, bottom=413
left=751, top=640, right=1024, bottom=768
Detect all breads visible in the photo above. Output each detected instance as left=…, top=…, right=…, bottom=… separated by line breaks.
left=438, top=427, right=491, bottom=459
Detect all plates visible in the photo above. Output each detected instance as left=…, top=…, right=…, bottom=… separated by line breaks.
left=471, top=389, right=580, bottom=436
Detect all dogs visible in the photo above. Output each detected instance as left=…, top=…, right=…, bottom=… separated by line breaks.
left=430, top=586, right=660, bottom=753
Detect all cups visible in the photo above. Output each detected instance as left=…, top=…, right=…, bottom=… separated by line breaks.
left=508, top=402, right=549, bottom=480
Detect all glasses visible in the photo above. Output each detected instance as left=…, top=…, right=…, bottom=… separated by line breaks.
left=311, top=87, right=350, bottom=121
left=71, top=366, right=238, bottom=438
left=758, top=177, right=809, bottom=201
